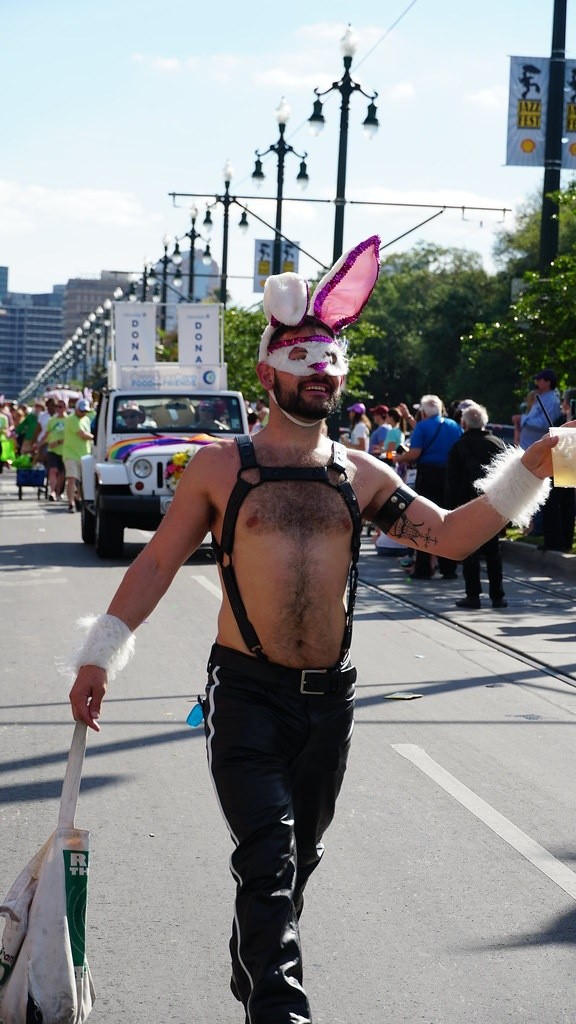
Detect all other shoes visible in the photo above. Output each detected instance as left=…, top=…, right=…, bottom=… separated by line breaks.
left=493, top=597, right=512, bottom=609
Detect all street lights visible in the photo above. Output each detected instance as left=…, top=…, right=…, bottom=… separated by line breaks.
left=147, top=234, right=183, bottom=344
left=171, top=204, right=212, bottom=302
left=252, top=96, right=308, bottom=274
left=308, top=25, right=380, bottom=264
left=15, top=260, right=161, bottom=404
left=203, top=161, right=249, bottom=310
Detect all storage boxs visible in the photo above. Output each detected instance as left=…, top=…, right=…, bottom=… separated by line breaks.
left=17, top=470, right=44, bottom=486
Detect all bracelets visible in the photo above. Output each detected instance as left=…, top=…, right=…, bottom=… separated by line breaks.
left=472, top=443, right=552, bottom=529
left=72, top=614, right=136, bottom=681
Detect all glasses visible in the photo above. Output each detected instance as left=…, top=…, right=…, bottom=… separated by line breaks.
left=201, top=408, right=212, bottom=412
left=123, top=413, right=137, bottom=418
left=55, top=405, right=63, bottom=408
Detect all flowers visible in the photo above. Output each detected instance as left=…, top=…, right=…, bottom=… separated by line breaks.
left=163, top=450, right=192, bottom=487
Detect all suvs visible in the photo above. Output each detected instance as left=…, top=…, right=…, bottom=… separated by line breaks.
left=80, top=388, right=251, bottom=559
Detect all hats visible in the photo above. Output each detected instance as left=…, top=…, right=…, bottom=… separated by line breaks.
left=124, top=404, right=144, bottom=414
left=76, top=399, right=92, bottom=412
left=347, top=402, right=366, bottom=414
left=200, top=400, right=216, bottom=410
left=534, top=369, right=556, bottom=383
left=369, top=405, right=390, bottom=414
left=36, top=401, right=46, bottom=411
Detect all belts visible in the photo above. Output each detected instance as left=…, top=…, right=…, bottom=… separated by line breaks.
left=211, top=645, right=358, bottom=694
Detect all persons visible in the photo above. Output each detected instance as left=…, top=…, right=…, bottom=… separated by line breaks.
left=326, top=394, right=508, bottom=608
left=185, top=400, right=232, bottom=430
left=113, top=400, right=155, bottom=434
left=245, top=398, right=269, bottom=434
left=69, top=235, right=576, bottom=1024
left=0, top=385, right=100, bottom=513
left=512, top=371, right=576, bottom=553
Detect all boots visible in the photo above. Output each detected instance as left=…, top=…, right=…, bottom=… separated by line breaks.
left=454, top=586, right=481, bottom=608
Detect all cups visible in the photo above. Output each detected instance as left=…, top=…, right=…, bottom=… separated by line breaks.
left=548, top=427, right=576, bottom=488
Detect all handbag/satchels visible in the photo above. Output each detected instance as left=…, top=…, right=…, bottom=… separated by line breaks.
left=0, top=722, right=95, bottom=1024
